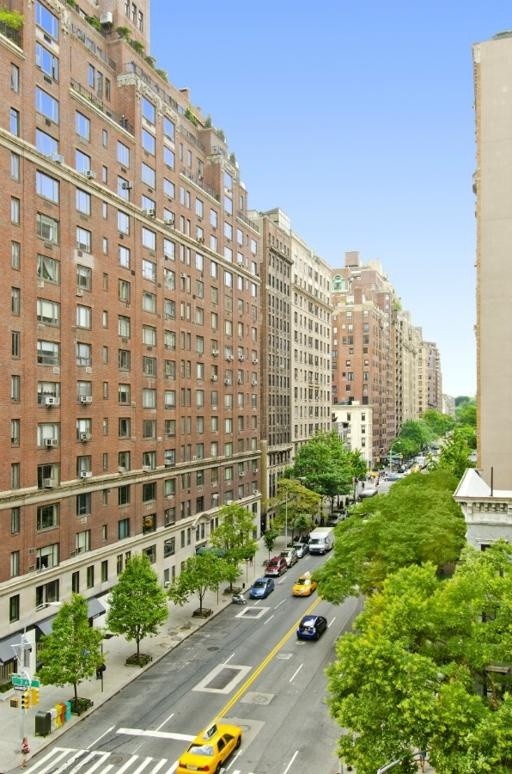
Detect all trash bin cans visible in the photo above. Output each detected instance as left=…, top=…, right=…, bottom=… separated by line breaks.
left=34, top=711, right=51, bottom=738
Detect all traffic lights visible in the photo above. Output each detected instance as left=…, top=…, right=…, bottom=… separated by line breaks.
left=32, top=688, right=40, bottom=705
left=21, top=690, right=31, bottom=710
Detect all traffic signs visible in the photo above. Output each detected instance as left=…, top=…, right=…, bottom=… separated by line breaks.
left=12, top=676, right=40, bottom=687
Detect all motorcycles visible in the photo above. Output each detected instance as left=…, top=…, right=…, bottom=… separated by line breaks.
left=232, top=592, right=247, bottom=604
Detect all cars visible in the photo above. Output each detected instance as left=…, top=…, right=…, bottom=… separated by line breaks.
left=177, top=721, right=243, bottom=774
left=324, top=485, right=379, bottom=525
left=382, top=440, right=440, bottom=481
left=292, top=571, right=318, bottom=597
left=249, top=576, right=275, bottom=600
left=265, top=536, right=309, bottom=576
left=297, top=615, right=328, bottom=640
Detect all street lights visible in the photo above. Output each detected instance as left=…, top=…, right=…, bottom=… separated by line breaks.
left=20, top=600, right=66, bottom=768
left=285, top=488, right=302, bottom=548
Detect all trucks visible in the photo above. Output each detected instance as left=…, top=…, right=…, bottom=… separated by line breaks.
left=308, top=526, right=335, bottom=554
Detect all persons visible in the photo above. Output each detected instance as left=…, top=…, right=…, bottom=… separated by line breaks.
left=20, top=737, right=31, bottom=769
